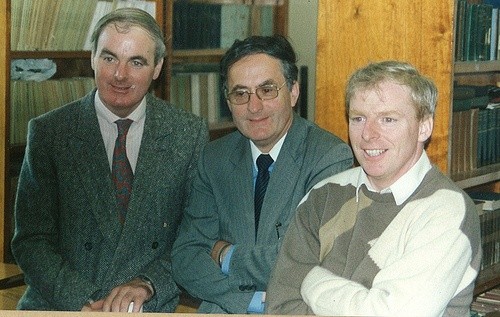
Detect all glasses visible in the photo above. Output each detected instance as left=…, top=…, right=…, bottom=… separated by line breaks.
left=223, top=81, right=286, bottom=105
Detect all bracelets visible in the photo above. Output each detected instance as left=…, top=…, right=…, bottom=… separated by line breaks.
left=217, top=242, right=232, bottom=268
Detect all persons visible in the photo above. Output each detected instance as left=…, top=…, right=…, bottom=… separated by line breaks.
left=170, top=33, right=353, bottom=313
left=262, top=60, right=482, bottom=316
left=10, top=8, right=207, bottom=313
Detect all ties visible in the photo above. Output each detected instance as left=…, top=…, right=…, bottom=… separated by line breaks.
left=111, top=118, right=133, bottom=224
left=254, top=154, right=274, bottom=241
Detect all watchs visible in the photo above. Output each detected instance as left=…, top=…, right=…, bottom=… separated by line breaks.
left=139, top=275, right=156, bottom=296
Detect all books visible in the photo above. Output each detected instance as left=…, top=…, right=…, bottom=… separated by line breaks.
left=451, top=0, right=500, bottom=317
left=9, top=0, right=275, bottom=147
